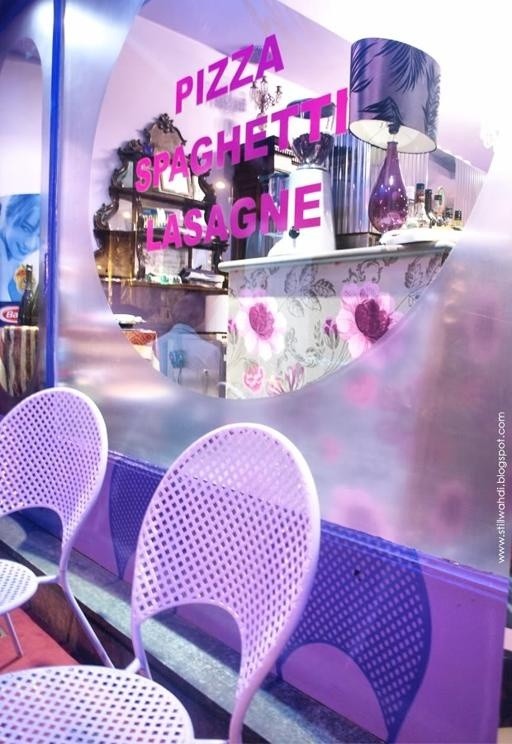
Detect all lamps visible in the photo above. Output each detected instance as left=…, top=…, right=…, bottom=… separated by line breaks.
left=346, top=35, right=443, bottom=236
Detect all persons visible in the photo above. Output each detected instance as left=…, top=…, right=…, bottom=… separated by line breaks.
left=145, top=287, right=222, bottom=404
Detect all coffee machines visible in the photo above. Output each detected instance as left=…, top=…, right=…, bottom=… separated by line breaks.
left=283, top=97, right=336, bottom=256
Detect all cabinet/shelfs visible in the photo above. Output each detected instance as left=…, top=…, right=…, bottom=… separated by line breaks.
left=91, top=110, right=229, bottom=295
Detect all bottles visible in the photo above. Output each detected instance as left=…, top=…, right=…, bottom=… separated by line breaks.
left=407, top=183, right=463, bottom=232
left=17, top=264, right=35, bottom=326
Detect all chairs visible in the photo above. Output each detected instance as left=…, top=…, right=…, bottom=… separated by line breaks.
left=1, top=382, right=324, bottom=744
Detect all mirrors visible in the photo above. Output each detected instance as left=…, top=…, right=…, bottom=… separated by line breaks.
left=84, top=1, right=495, bottom=399
